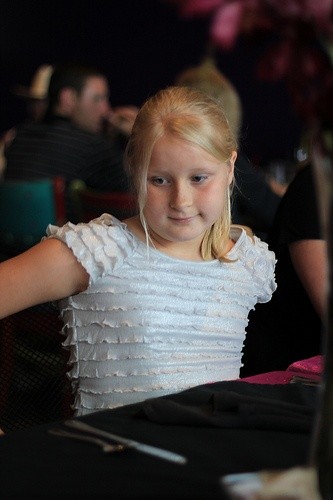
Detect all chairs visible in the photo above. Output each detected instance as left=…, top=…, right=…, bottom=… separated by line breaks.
left=0, top=174, right=128, bottom=256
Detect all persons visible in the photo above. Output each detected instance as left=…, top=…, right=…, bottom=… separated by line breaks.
left=0, top=57, right=332, bottom=419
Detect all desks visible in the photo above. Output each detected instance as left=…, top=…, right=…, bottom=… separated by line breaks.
left=1, top=358, right=325, bottom=499
